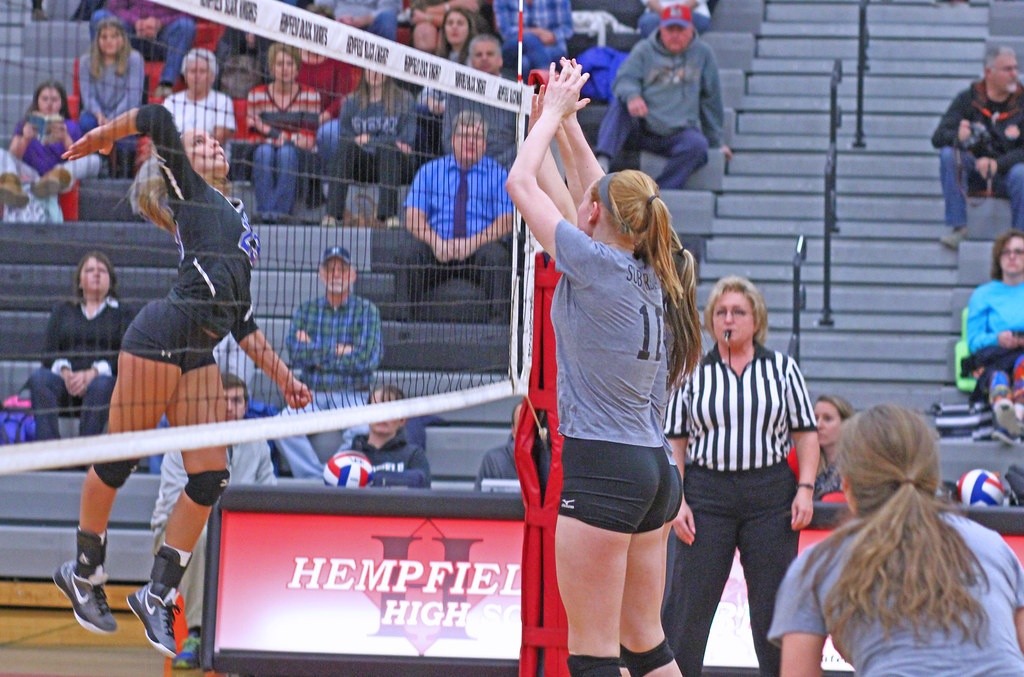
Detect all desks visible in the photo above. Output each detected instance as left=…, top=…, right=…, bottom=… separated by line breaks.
left=206, top=482, right=1024, bottom=677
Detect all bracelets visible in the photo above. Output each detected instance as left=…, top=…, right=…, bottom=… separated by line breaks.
left=796, top=483, right=814, bottom=491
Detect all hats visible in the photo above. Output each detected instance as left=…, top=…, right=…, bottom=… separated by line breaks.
left=321, top=245, right=351, bottom=266
left=661, top=4, right=692, bottom=28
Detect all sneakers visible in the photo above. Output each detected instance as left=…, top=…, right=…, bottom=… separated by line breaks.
left=126, top=582, right=181, bottom=659
left=53, top=559, right=119, bottom=635
left=172, top=634, right=200, bottom=670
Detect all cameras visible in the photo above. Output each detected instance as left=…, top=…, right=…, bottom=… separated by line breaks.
left=964, top=120, right=992, bottom=151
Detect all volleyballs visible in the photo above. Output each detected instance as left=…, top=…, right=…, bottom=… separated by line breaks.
left=954, top=468, right=1004, bottom=505
left=323, top=450, right=375, bottom=490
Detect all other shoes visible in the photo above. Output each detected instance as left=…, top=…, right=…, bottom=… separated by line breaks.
left=995, top=397, right=1024, bottom=437
left=153, top=85, right=172, bottom=97
left=260, top=211, right=283, bottom=225
left=31, top=163, right=71, bottom=197
left=940, top=227, right=969, bottom=251
left=0, top=173, right=29, bottom=209
left=991, top=425, right=1022, bottom=446
left=386, top=216, right=403, bottom=230
left=319, top=214, right=336, bottom=226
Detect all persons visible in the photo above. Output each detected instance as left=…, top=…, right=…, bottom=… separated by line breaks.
left=0, top=1, right=1024, bottom=677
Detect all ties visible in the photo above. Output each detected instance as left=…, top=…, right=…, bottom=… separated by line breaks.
left=452, top=169, right=470, bottom=238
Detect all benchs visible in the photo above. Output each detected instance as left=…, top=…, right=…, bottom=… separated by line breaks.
left=0, top=0, right=1024, bottom=582
